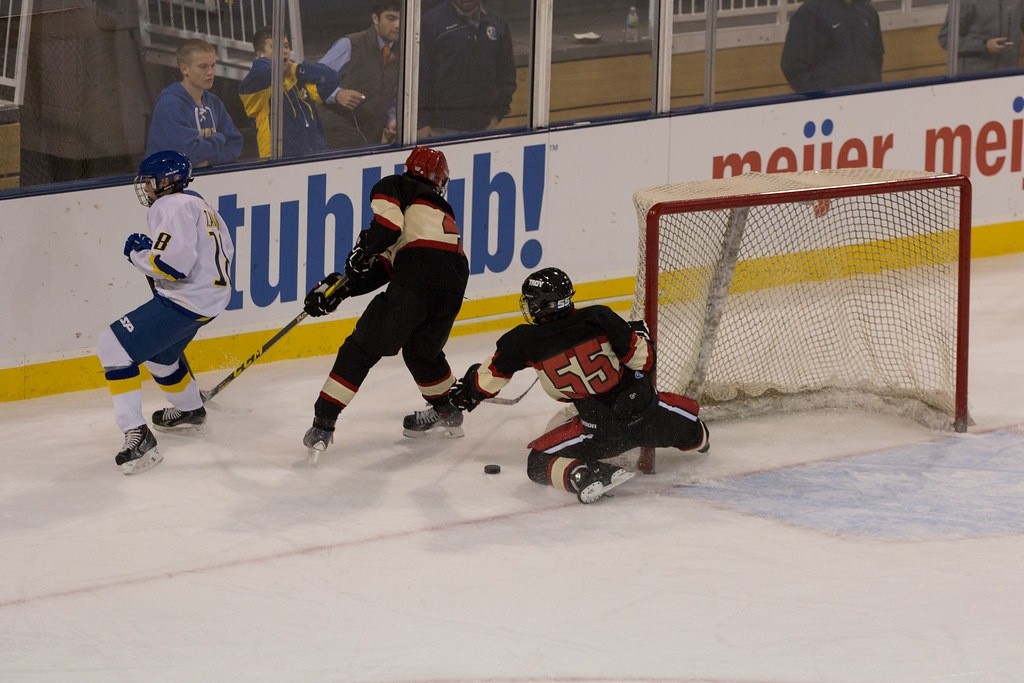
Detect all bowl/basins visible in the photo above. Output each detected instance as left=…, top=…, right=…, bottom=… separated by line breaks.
left=574, top=31, right=601, bottom=44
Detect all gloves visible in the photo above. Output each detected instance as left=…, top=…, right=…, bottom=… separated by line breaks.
left=447, top=364, right=500, bottom=414
left=346, top=243, right=370, bottom=281
left=628, top=320, right=650, bottom=341
left=304, top=271, right=349, bottom=318
left=124, top=232, right=154, bottom=257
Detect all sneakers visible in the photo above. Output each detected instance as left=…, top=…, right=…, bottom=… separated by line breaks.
left=404, top=378, right=465, bottom=438
left=151, top=406, right=207, bottom=433
left=115, top=423, right=164, bottom=475
left=303, top=417, right=336, bottom=464
left=568, top=455, right=637, bottom=504
left=696, top=420, right=710, bottom=455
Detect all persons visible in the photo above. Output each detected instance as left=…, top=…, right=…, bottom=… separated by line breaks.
left=302, top=146, right=470, bottom=467
left=97, top=149, right=234, bottom=475
left=416, top=0, right=519, bottom=141
left=239, top=25, right=343, bottom=160
left=938, top=0, right=1024, bottom=73
left=451, top=267, right=711, bottom=503
left=314, top=0, right=404, bottom=146
left=779, top=0, right=884, bottom=94
left=144, top=38, right=244, bottom=169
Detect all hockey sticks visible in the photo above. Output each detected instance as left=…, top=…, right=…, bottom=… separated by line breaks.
left=481, top=376, right=539, bottom=406
left=199, top=200, right=411, bottom=404
left=145, top=274, right=207, bottom=404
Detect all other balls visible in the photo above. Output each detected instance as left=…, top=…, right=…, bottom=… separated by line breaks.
left=484, top=465, right=500, bottom=474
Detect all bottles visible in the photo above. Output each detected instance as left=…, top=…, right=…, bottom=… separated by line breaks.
left=626, top=7, right=640, bottom=43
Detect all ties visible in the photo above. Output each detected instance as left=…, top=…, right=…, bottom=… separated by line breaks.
left=383, top=44, right=389, bottom=67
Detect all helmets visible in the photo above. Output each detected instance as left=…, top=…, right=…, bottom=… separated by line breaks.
left=519, top=266, right=576, bottom=324
left=133, top=149, right=194, bottom=207
left=404, top=145, right=450, bottom=197
